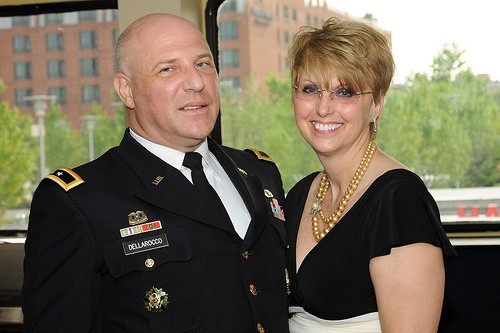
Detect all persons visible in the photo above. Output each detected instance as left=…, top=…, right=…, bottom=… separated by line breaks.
left=281, top=17, right=458, bottom=333
left=21, top=12, right=296, bottom=333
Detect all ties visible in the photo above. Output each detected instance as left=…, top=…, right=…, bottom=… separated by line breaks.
left=181, top=151, right=236, bottom=236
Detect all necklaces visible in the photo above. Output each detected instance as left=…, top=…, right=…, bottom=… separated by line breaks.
left=316, top=139, right=372, bottom=224
left=310, top=143, right=378, bottom=243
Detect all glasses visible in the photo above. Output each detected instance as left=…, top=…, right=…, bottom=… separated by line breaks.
left=292, top=85, right=379, bottom=104
